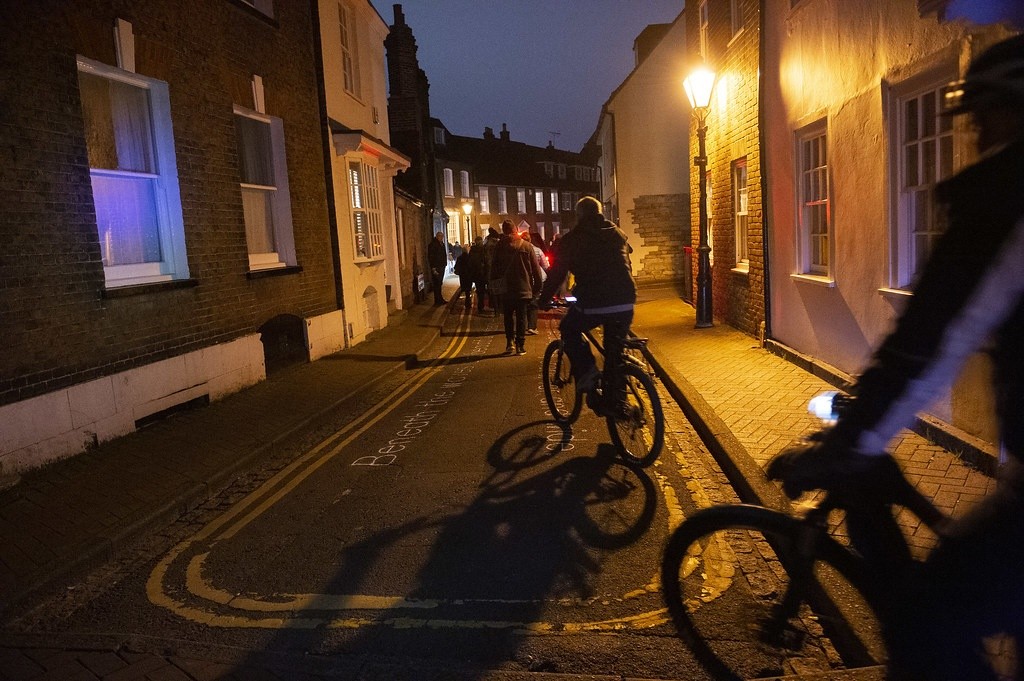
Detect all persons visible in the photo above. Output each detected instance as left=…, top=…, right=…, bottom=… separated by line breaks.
left=766, top=33, right=1024, bottom=681
left=428, top=231, right=447, bottom=303
left=446, top=218, right=566, bottom=355
left=536, top=196, right=639, bottom=422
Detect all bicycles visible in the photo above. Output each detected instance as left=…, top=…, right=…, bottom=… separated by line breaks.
left=658, top=390, right=1024, bottom=681
left=527, top=296, right=666, bottom=470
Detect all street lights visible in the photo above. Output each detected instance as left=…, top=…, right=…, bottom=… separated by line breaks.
left=590, top=165, right=603, bottom=203
left=462, top=202, right=473, bottom=247
left=681, top=53, right=717, bottom=330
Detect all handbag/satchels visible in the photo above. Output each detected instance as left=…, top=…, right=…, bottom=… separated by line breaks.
left=488, top=278, right=507, bottom=300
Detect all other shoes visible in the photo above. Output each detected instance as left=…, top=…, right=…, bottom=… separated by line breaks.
left=516, top=348, right=527, bottom=355
left=506, top=342, right=515, bottom=353
left=528, top=328, right=539, bottom=334
left=435, top=299, right=448, bottom=304
left=577, top=369, right=601, bottom=393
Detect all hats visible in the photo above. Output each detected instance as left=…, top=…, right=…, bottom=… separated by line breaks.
left=488, top=228, right=499, bottom=238
